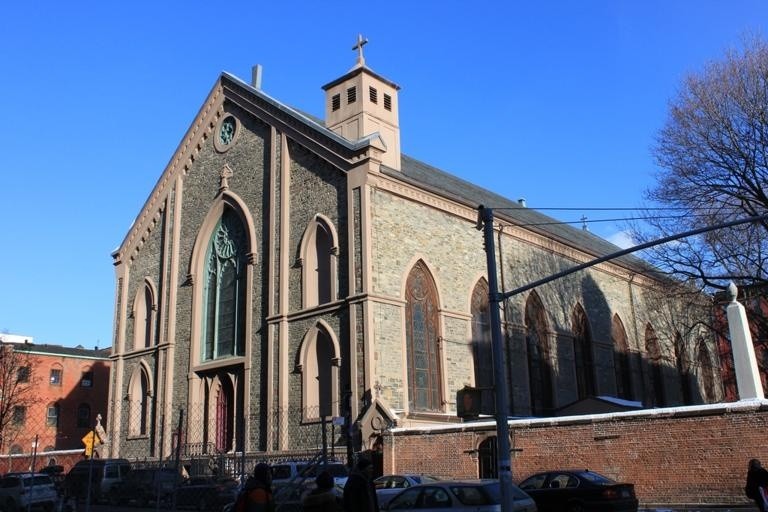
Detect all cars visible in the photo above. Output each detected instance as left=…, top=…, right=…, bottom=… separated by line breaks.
left=0, top=457, right=238, bottom=511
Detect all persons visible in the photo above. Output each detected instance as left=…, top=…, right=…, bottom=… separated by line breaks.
left=301, top=469, right=341, bottom=511
left=241, top=462, right=277, bottom=511
left=339, top=453, right=381, bottom=512
left=744, top=458, right=768, bottom=512
